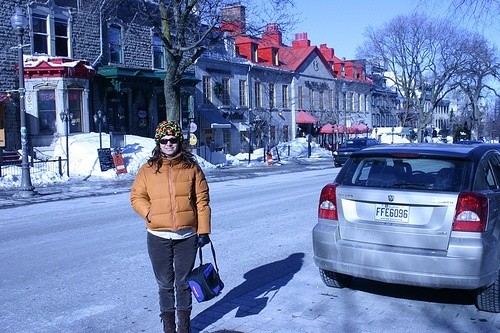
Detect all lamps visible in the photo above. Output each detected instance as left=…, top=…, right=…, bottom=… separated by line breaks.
left=278, top=110, right=283, bottom=116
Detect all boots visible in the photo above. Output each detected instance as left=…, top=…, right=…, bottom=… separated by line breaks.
left=160, top=311, right=176, bottom=333
left=177, top=310, right=190, bottom=333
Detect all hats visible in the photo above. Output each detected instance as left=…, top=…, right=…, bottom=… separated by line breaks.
left=155, top=120, right=183, bottom=140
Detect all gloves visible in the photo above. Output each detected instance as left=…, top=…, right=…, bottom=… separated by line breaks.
left=197, top=234, right=210, bottom=247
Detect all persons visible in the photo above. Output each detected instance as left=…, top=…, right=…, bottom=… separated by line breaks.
left=409, top=128, right=437, bottom=144
left=392, top=160, right=405, bottom=171
left=130, top=120, right=211, bottom=333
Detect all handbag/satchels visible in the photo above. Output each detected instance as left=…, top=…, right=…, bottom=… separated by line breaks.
left=188, top=237, right=224, bottom=302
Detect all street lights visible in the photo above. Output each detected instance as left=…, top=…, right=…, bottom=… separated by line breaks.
left=60, top=105, right=74, bottom=177
left=93, top=108, right=107, bottom=149
left=9, top=10, right=41, bottom=200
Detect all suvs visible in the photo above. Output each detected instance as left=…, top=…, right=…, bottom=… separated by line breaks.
left=312, top=140, right=500, bottom=313
left=332, top=137, right=379, bottom=168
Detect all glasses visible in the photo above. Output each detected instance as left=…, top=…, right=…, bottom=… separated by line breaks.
left=159, top=137, right=179, bottom=144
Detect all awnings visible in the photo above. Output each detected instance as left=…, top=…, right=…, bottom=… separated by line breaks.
left=295, top=110, right=317, bottom=123
left=319, top=123, right=370, bottom=134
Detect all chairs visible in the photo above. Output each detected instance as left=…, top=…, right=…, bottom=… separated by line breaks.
left=365, top=159, right=454, bottom=191
